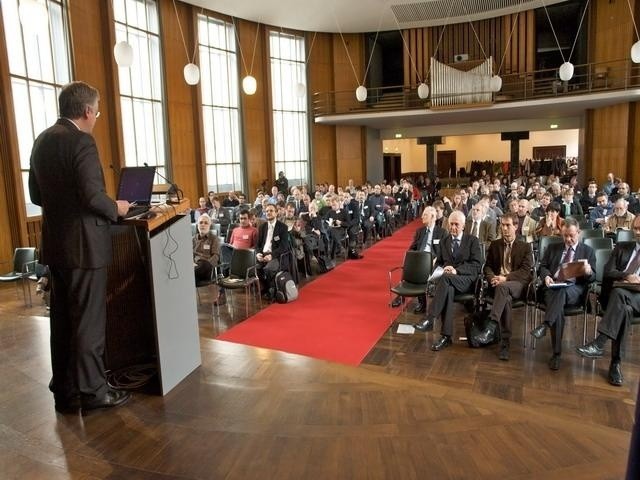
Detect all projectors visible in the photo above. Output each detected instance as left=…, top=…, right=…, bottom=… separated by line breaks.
left=454, top=53, right=473, bottom=62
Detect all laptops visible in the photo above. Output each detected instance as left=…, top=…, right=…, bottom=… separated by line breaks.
left=116, top=165, right=157, bottom=218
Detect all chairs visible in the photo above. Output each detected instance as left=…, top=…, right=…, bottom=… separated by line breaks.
left=190, top=183, right=440, bottom=330
left=0, top=246, right=50, bottom=306
left=387, top=206, right=634, bottom=360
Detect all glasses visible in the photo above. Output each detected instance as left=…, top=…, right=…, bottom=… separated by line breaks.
left=90, top=106, right=100, bottom=117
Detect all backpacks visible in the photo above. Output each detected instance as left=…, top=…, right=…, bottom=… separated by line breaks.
left=275, top=271, right=298, bottom=303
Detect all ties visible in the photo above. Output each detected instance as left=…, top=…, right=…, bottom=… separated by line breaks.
left=420, top=229, right=429, bottom=252
left=564, top=247, right=572, bottom=263
left=472, top=222, right=477, bottom=237
left=626, top=249, right=640, bottom=274
left=453, top=240, right=458, bottom=259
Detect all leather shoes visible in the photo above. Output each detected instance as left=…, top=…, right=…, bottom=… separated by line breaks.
left=413, top=304, right=434, bottom=331
left=82, top=390, right=131, bottom=409
left=530, top=326, right=546, bottom=338
left=576, top=342, right=604, bottom=357
left=388, top=296, right=405, bottom=307
left=431, top=335, right=452, bottom=350
left=550, top=356, right=559, bottom=370
left=474, top=329, right=510, bottom=359
left=609, top=362, right=623, bottom=385
left=214, top=293, right=226, bottom=305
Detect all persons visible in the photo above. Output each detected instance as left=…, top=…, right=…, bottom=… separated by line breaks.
left=34, top=265, right=53, bottom=312
left=28, top=81, right=133, bottom=416
left=190, top=158, right=639, bottom=387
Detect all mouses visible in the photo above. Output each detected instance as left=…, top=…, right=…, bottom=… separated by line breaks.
left=140, top=210, right=157, bottom=219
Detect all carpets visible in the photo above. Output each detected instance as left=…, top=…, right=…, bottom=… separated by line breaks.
left=213, top=216, right=426, bottom=368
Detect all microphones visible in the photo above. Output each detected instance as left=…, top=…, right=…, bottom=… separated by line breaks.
left=144, top=162, right=181, bottom=205
left=109, top=164, right=120, bottom=175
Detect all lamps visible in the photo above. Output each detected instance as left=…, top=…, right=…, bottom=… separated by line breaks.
left=323, top=0, right=591, bottom=103
left=172, top=0, right=323, bottom=97
left=628, top=0, right=640, bottom=66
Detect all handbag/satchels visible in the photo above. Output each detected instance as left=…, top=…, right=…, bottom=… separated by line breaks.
left=317, top=255, right=334, bottom=272
left=465, top=310, right=498, bottom=347
